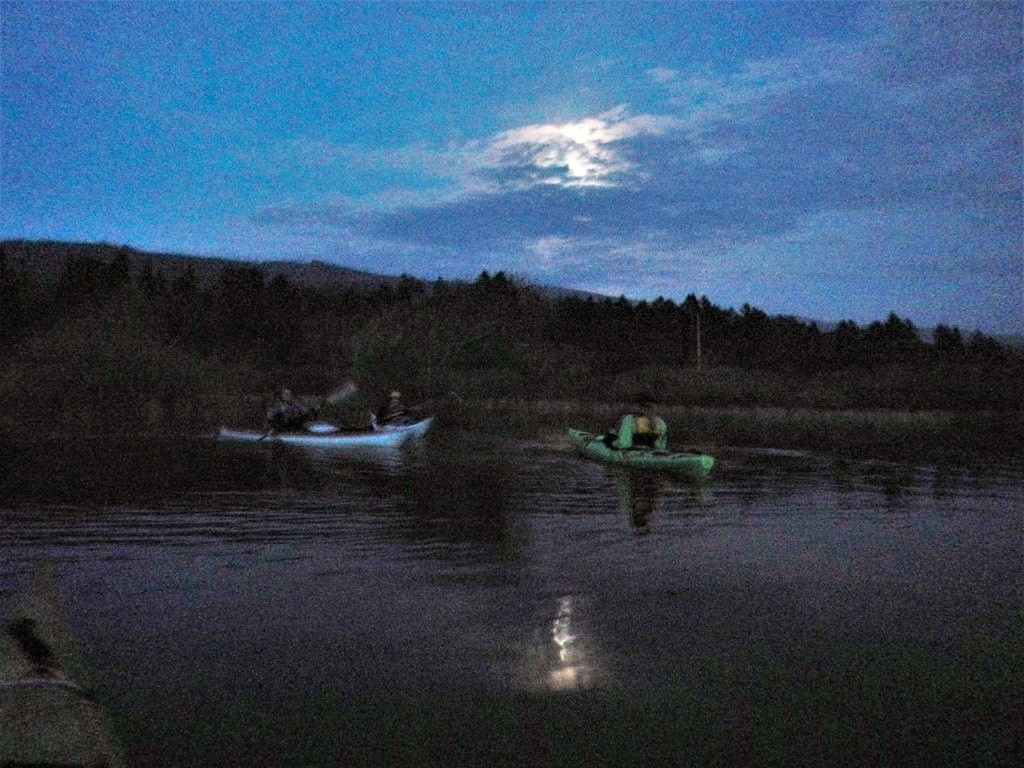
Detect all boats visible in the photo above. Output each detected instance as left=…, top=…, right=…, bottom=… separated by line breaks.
left=210, top=416, right=436, bottom=447
left=568, top=427, right=714, bottom=474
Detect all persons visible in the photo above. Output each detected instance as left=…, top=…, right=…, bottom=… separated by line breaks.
left=267, top=387, right=317, bottom=432
left=604, top=399, right=668, bottom=451
left=377, top=391, right=416, bottom=424
